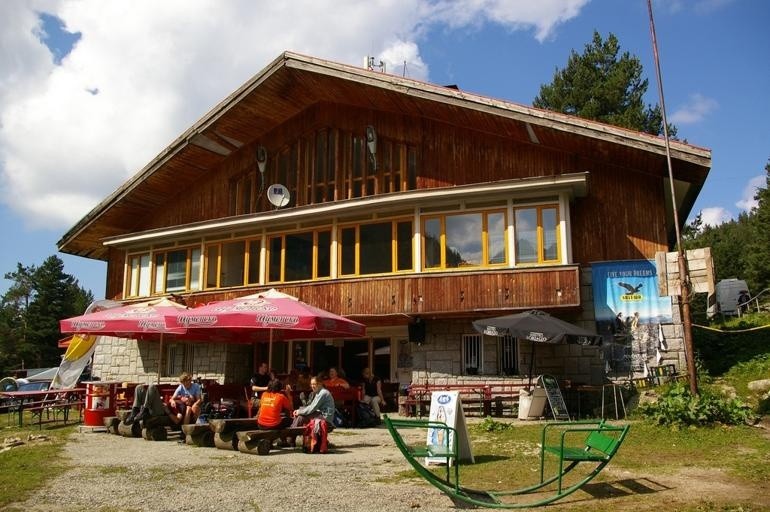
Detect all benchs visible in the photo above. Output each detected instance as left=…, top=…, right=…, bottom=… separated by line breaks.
left=0, top=386, right=127, bottom=430
left=103, top=416, right=309, bottom=456
left=404, top=383, right=544, bottom=418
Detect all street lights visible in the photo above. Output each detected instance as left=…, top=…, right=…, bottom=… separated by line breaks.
left=18, top=313, right=27, bottom=370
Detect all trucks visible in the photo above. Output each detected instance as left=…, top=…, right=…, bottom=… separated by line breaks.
left=706, top=278, right=752, bottom=323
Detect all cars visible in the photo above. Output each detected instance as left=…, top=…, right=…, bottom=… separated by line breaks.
left=10, top=382, right=51, bottom=409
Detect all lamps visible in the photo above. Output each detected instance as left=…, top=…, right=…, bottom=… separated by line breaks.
left=255, top=145, right=268, bottom=192
left=366, top=125, right=377, bottom=170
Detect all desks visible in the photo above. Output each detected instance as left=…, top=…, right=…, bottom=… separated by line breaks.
left=115, top=408, right=131, bottom=420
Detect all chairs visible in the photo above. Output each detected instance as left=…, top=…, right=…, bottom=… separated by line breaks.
left=244, top=385, right=257, bottom=417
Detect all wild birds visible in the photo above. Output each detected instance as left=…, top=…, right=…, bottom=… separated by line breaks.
left=618, top=282, right=643, bottom=295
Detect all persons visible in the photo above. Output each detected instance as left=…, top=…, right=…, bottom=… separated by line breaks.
left=250, top=361, right=351, bottom=409
left=122, top=383, right=196, bottom=426
left=360, top=366, right=387, bottom=424
left=169, top=372, right=203, bottom=443
left=738, top=290, right=752, bottom=313
left=257, top=378, right=293, bottom=447
left=609, top=312, right=640, bottom=335
left=287, top=375, right=336, bottom=447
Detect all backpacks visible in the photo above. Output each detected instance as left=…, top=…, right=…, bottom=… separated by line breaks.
left=356, top=399, right=379, bottom=428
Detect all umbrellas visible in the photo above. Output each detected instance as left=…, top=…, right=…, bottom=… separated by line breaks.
left=57, top=296, right=194, bottom=384
left=177, top=286, right=367, bottom=374
left=471, top=309, right=604, bottom=392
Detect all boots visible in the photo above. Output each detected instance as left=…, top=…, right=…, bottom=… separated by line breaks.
left=125, top=407, right=139, bottom=424
left=134, top=405, right=150, bottom=422
